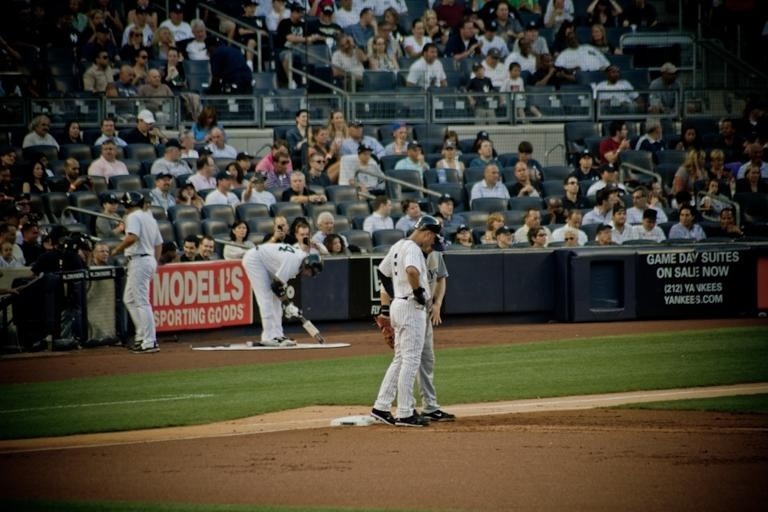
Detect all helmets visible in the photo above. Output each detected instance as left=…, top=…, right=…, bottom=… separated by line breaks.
left=303, top=254, right=324, bottom=278
left=119, top=191, right=144, bottom=208
left=416, top=215, right=441, bottom=233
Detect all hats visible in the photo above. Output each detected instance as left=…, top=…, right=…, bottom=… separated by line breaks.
left=236, top=150, right=254, bottom=161
left=496, top=224, right=514, bottom=236
left=138, top=109, right=187, bottom=149
left=457, top=223, right=470, bottom=234
left=137, top=2, right=335, bottom=23
left=484, top=19, right=499, bottom=35
left=472, top=47, right=502, bottom=73
left=346, top=121, right=495, bottom=205
left=596, top=222, right=613, bottom=233
left=98, top=193, right=120, bottom=205
left=522, top=17, right=539, bottom=31
left=215, top=169, right=232, bottom=181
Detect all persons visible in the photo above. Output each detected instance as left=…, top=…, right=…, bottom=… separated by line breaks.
left=1, top=0, right=768, bottom=355
left=378, top=235, right=457, bottom=421
left=371, top=214, right=442, bottom=426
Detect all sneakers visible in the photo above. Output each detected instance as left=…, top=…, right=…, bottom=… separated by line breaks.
left=420, top=409, right=456, bottom=422
left=371, top=408, right=394, bottom=426
left=396, top=409, right=431, bottom=428
left=127, top=339, right=161, bottom=354
left=261, top=335, right=297, bottom=348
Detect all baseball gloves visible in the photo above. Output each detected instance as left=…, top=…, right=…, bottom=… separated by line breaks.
left=376, top=314, right=394, bottom=349
